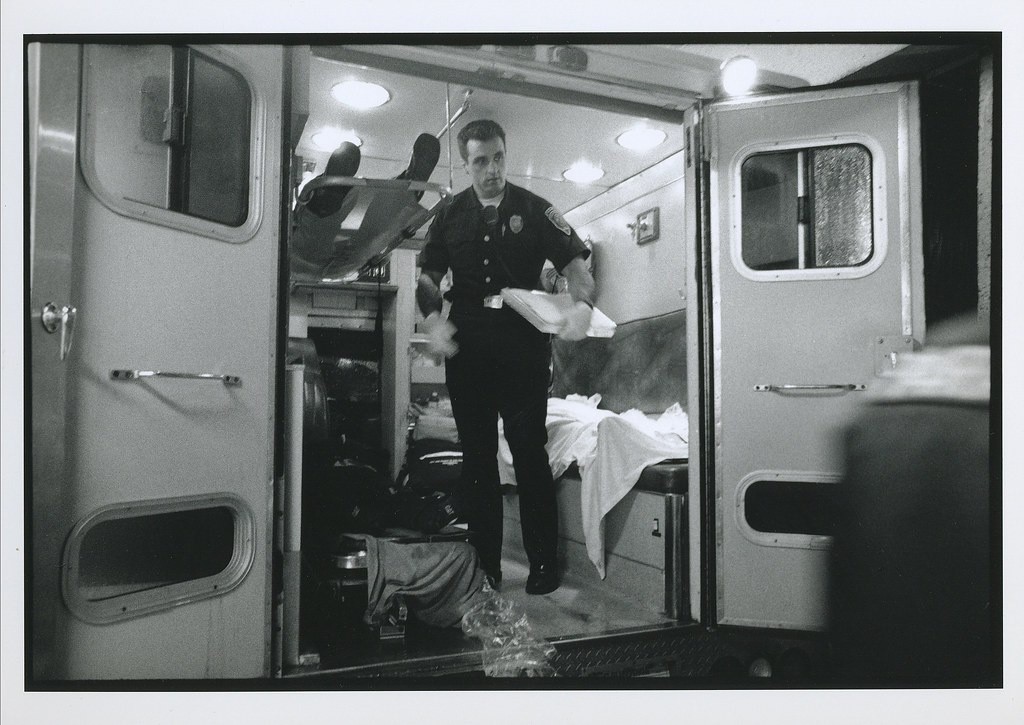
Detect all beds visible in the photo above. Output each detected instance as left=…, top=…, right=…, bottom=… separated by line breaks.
left=292, top=174, right=455, bottom=284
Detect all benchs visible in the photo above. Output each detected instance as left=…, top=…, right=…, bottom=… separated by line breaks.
left=407, top=308, right=689, bottom=620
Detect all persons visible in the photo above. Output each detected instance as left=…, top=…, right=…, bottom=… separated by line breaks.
left=283, top=127, right=440, bottom=280
left=417, top=119, right=596, bottom=594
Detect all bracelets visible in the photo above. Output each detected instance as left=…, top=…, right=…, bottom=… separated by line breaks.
left=576, top=298, right=593, bottom=310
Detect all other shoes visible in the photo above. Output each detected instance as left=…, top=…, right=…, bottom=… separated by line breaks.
left=405, top=133, right=440, bottom=201
left=317, top=141, right=361, bottom=206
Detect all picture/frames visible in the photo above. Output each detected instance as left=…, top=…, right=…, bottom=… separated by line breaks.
left=636, top=207, right=659, bottom=245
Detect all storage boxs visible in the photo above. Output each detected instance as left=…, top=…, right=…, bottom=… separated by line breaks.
left=312, top=292, right=356, bottom=310
left=357, top=295, right=388, bottom=311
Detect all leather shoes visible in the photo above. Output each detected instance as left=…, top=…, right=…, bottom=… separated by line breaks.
left=487, top=571, right=502, bottom=586
left=526, top=569, right=560, bottom=594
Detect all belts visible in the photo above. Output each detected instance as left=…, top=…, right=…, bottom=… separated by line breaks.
left=475, top=294, right=509, bottom=309
